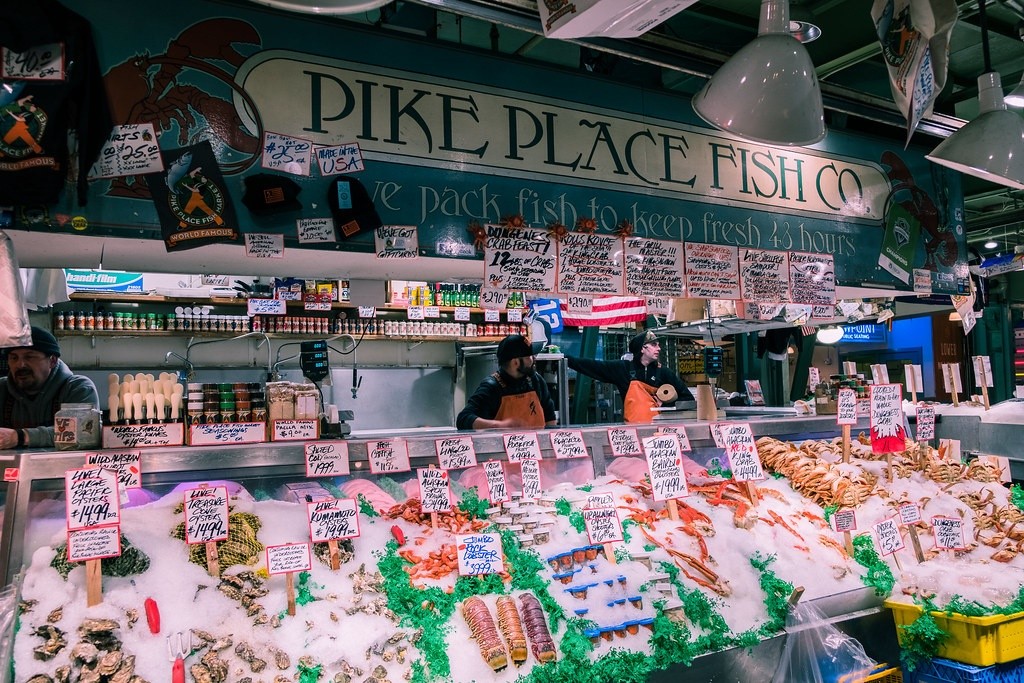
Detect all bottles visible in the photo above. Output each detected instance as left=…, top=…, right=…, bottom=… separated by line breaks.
left=829, top=374, right=870, bottom=397
left=330, top=312, right=384, bottom=335
left=428, top=282, right=482, bottom=308
left=187, top=382, right=266, bottom=424
left=167, top=306, right=328, bottom=333
left=384, top=318, right=529, bottom=337
left=54, top=308, right=165, bottom=331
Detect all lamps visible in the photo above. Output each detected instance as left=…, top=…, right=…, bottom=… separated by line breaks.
left=924, top=0, right=1024, bottom=190
left=984, top=232, right=998, bottom=249
left=1011, top=225, right=1024, bottom=272
left=691, top=0, right=828, bottom=147
left=1004, top=74, right=1024, bottom=106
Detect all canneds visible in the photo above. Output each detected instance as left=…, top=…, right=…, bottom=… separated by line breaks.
left=113, top=307, right=250, bottom=332
left=252, top=315, right=527, bottom=337
left=824, top=374, right=873, bottom=403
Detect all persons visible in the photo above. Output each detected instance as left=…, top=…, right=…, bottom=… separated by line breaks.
left=566, top=330, right=695, bottom=423
left=456, top=335, right=557, bottom=430
left=0, top=327, right=102, bottom=450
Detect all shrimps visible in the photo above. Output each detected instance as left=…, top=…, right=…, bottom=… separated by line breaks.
left=461, top=595, right=509, bottom=673
left=517, top=592, right=558, bottom=665
left=495, top=594, right=528, bottom=669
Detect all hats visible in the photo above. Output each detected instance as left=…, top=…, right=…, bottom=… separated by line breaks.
left=496, top=334, right=538, bottom=365
left=0, top=326, right=61, bottom=357
left=628, top=331, right=664, bottom=354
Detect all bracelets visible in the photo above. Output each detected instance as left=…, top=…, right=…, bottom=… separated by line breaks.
left=14, top=429, right=25, bottom=450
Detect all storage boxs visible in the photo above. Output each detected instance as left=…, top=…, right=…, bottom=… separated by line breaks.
left=807, top=601, right=1024, bottom=683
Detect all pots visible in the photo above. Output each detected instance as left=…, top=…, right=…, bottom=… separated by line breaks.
left=233, top=278, right=273, bottom=299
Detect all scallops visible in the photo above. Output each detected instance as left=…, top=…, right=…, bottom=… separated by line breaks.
left=16, top=597, right=151, bottom=683
left=187, top=562, right=425, bottom=683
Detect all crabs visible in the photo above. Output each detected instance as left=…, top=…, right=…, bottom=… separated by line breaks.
left=754, top=430, right=1024, bottom=565
